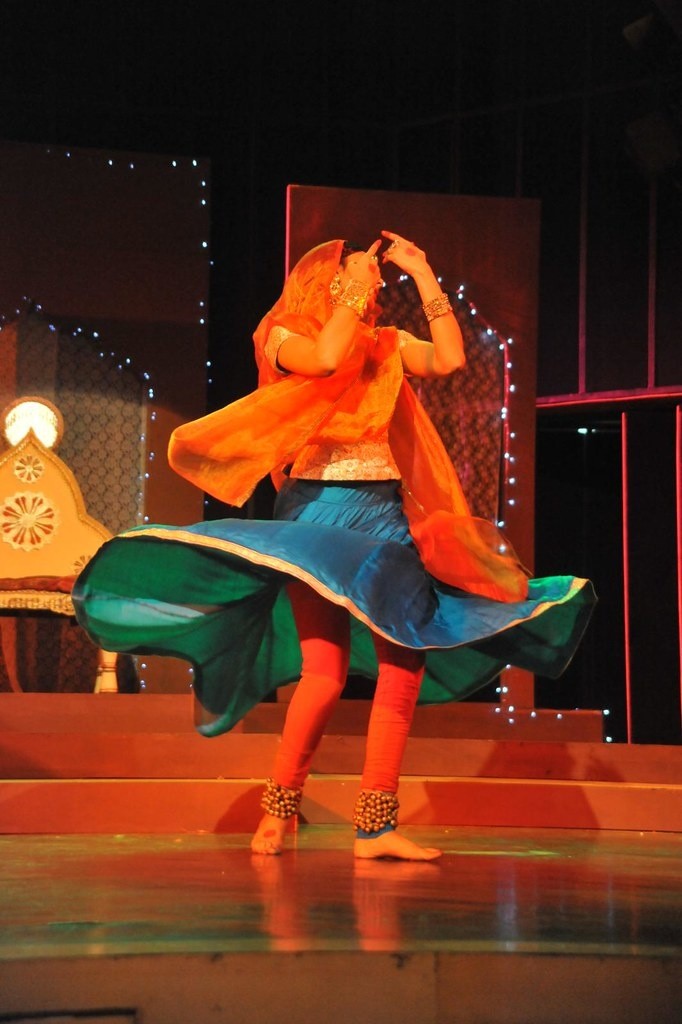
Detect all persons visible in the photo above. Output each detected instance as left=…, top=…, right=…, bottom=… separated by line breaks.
left=250, top=855, right=442, bottom=951
left=71, top=230, right=597, bottom=859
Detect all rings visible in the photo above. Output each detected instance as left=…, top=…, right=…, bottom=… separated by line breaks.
left=392, top=238, right=399, bottom=248
left=371, top=255, right=378, bottom=260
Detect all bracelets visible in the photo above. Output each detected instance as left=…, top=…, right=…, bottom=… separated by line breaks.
left=423, top=293, right=452, bottom=322
left=340, top=278, right=376, bottom=316
